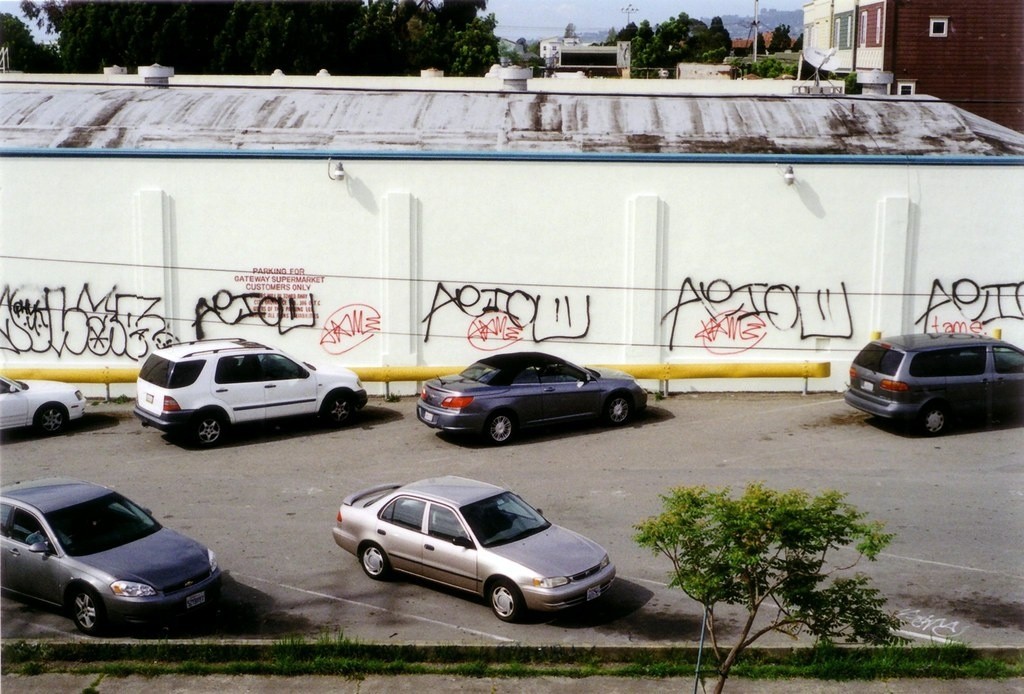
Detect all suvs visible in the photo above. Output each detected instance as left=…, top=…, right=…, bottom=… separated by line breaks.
left=132, top=337, right=368, bottom=450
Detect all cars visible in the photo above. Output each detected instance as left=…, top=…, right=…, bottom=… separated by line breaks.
left=844, top=333, right=1024, bottom=438
left=333, top=474, right=616, bottom=623
left=0, top=375, right=89, bottom=438
left=0, top=476, right=222, bottom=636
left=416, top=352, right=647, bottom=447
